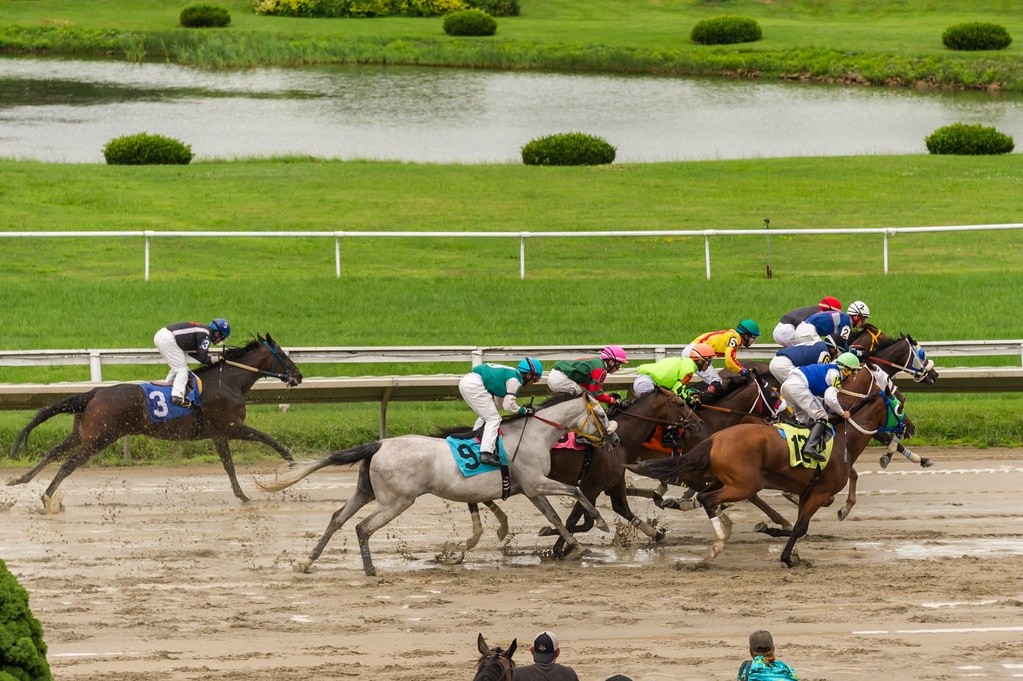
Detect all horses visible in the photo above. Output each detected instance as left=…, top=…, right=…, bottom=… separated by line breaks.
left=472, top=631, right=517, bottom=681
left=253, top=391, right=620, bottom=576
left=5, top=330, right=302, bottom=512
left=427, top=323, right=938, bottom=567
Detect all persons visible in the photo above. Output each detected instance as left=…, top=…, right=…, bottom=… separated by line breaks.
left=514, top=631, right=580, bottom=681
left=769, top=335, right=846, bottom=385
left=605, top=675, right=633, bottom=681
left=737, top=631, right=799, bottom=681
left=633, top=343, right=715, bottom=448
left=681, top=319, right=762, bottom=400
left=772, top=297, right=842, bottom=348
left=154, top=318, right=231, bottom=408
left=459, top=356, right=543, bottom=465
left=547, top=346, right=629, bottom=445
left=781, top=352, right=863, bottom=461
left=795, top=301, right=872, bottom=358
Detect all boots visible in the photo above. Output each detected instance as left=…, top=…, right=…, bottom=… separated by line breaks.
left=803, top=416, right=828, bottom=463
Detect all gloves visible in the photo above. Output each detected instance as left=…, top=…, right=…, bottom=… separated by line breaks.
left=741, top=367, right=750, bottom=377
left=219, top=355, right=225, bottom=363
left=685, top=395, right=696, bottom=406
left=613, top=397, right=621, bottom=406
left=524, top=407, right=534, bottom=415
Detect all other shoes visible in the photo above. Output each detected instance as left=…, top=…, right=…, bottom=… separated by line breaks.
left=172, top=396, right=192, bottom=410
left=662, top=438, right=680, bottom=447
left=576, top=439, right=591, bottom=445
left=481, top=452, right=501, bottom=466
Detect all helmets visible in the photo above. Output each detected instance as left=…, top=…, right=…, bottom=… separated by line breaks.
left=736, top=318, right=761, bottom=337
left=518, top=356, right=543, bottom=380
left=600, top=342, right=628, bottom=364
left=824, top=333, right=845, bottom=351
left=209, top=318, right=231, bottom=340
left=848, top=301, right=870, bottom=318
left=838, top=352, right=861, bottom=371
left=820, top=297, right=843, bottom=312
left=690, top=344, right=717, bottom=359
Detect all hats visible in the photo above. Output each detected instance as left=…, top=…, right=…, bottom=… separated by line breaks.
left=749, top=631, right=773, bottom=653
left=533, top=629, right=558, bottom=664
